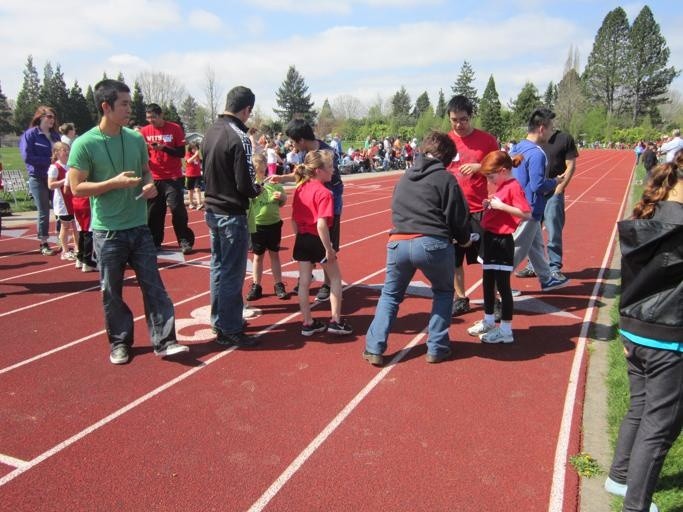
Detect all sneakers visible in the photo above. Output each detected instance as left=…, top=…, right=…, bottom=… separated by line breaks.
left=511, top=267, right=568, bottom=296
left=314, top=284, right=329, bottom=301
left=60, top=249, right=98, bottom=272
left=159, top=344, right=189, bottom=356
left=301, top=318, right=326, bottom=336
left=466, top=298, right=513, bottom=344
left=212, top=305, right=256, bottom=348
left=426, top=350, right=451, bottom=363
left=180, top=239, right=192, bottom=253
left=246, top=284, right=261, bottom=301
left=451, top=297, right=468, bottom=315
left=604, top=477, right=657, bottom=512
left=327, top=320, right=351, bottom=334
left=39, top=247, right=54, bottom=256
left=362, top=351, right=382, bottom=365
left=188, top=203, right=203, bottom=210
left=273, top=282, right=289, bottom=299
left=109, top=346, right=129, bottom=364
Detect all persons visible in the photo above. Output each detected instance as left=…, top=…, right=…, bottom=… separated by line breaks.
left=362, top=132, right=472, bottom=369
left=246, top=153, right=289, bottom=301
left=283, top=120, right=343, bottom=304
left=511, top=109, right=569, bottom=289
left=289, top=151, right=352, bottom=337
left=16, top=103, right=206, bottom=274
left=65, top=80, right=192, bottom=364
left=248, top=127, right=420, bottom=180
left=441, top=93, right=498, bottom=317
left=467, top=151, right=532, bottom=347
left=513, top=130, right=577, bottom=277
left=198, top=84, right=266, bottom=349
left=578, top=130, right=682, bottom=174
left=604, top=147, right=682, bottom=511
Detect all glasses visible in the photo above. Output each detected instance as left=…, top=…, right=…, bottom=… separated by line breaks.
left=42, top=115, right=55, bottom=119
